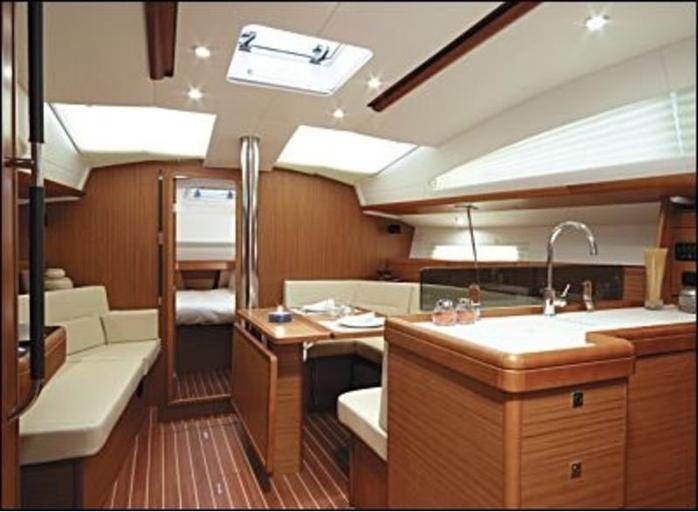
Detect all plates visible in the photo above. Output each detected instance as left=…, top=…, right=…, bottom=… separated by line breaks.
left=338, top=317, right=386, bottom=327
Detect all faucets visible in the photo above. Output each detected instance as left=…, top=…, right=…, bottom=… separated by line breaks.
left=540, top=218, right=598, bottom=314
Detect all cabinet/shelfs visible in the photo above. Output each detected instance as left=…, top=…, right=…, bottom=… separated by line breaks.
left=385, top=318, right=635, bottom=510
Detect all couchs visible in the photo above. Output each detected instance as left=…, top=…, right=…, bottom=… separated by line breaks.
left=8, top=286, right=165, bottom=509
left=283, top=281, right=543, bottom=413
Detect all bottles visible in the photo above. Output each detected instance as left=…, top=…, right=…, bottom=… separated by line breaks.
left=455, top=298, right=478, bottom=324
left=431, top=297, right=457, bottom=325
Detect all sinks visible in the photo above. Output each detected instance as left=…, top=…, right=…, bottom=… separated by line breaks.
left=557, top=307, right=696, bottom=330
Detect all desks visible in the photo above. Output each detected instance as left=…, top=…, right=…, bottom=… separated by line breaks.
left=228, top=301, right=389, bottom=477
